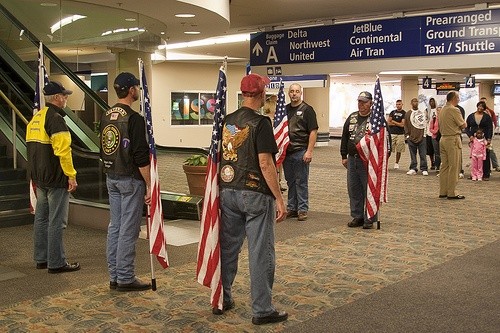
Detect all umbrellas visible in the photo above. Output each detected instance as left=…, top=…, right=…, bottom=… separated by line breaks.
left=196, top=67, right=227, bottom=311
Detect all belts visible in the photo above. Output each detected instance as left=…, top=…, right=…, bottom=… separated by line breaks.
left=349, top=154, right=360, bottom=159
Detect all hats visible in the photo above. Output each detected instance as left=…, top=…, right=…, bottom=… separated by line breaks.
left=112, top=72, right=140, bottom=90
left=43, top=80, right=73, bottom=95
left=240, top=74, right=270, bottom=96
left=357, top=91, right=372, bottom=102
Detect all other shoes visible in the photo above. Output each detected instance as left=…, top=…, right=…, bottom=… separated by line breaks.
left=458, top=173, right=464, bottom=179
left=394, top=162, right=399, bottom=169
left=472, top=178, right=482, bottom=181
left=484, top=177, right=489, bottom=181
left=495, top=166, right=500, bottom=172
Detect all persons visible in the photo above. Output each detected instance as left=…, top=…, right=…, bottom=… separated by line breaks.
left=464, top=98, right=500, bottom=181
left=425, top=98, right=443, bottom=176
left=26, top=82, right=81, bottom=274
left=439, top=92, right=465, bottom=200
left=385, top=100, right=406, bottom=168
left=99, top=73, right=151, bottom=290
left=340, top=91, right=392, bottom=228
left=404, top=98, right=429, bottom=176
left=282, top=84, right=318, bottom=222
left=213, top=74, right=288, bottom=325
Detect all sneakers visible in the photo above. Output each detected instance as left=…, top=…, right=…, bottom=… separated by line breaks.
left=422, top=171, right=428, bottom=176
left=407, top=170, right=416, bottom=175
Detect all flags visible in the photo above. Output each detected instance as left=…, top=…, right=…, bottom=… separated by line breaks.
left=29, top=49, right=50, bottom=215
left=272, top=83, right=290, bottom=169
left=138, top=61, right=169, bottom=271
left=355, top=75, right=391, bottom=222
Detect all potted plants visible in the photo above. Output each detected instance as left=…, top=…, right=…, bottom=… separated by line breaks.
left=183, top=154, right=208, bottom=195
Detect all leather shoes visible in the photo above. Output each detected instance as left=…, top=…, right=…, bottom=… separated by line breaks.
left=110, top=277, right=151, bottom=291
left=447, top=195, right=465, bottom=200
left=363, top=223, right=373, bottom=229
left=48, top=262, right=81, bottom=274
left=298, top=211, right=307, bottom=221
left=347, top=217, right=364, bottom=227
left=252, top=311, right=288, bottom=325
left=36, top=262, right=48, bottom=269
left=439, top=195, right=447, bottom=198
left=286, top=211, right=298, bottom=218
left=213, top=301, right=235, bottom=315
